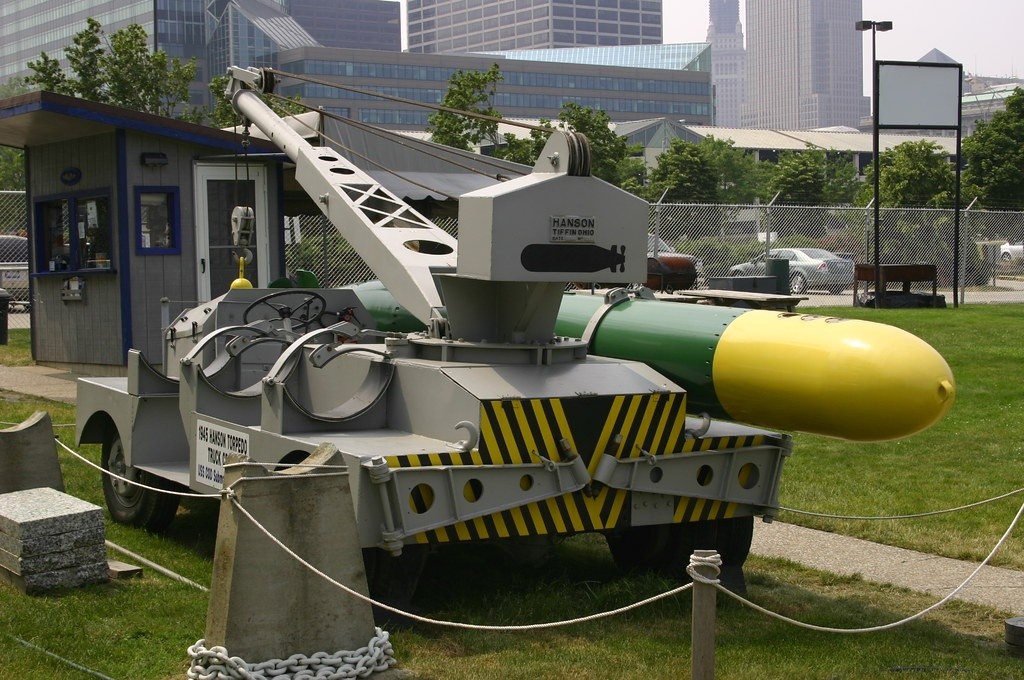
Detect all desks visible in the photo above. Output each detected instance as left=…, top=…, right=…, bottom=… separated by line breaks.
left=678, top=290, right=810, bottom=312
left=653, top=293, right=707, bottom=304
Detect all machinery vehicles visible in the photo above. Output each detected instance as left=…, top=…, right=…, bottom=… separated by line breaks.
left=72, top=58, right=957, bottom=590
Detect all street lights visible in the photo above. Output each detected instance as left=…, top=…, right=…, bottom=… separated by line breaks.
left=855, top=20, right=894, bottom=308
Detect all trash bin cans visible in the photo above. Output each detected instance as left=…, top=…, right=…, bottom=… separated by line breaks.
left=766, top=258, right=790, bottom=310
left=0, top=288, right=14, bottom=345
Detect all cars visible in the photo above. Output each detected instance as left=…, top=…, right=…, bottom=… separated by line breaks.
left=729, top=247, right=856, bottom=295
left=648, top=234, right=705, bottom=303
left=1001, top=239, right=1024, bottom=262
left=0, top=235, right=30, bottom=314
